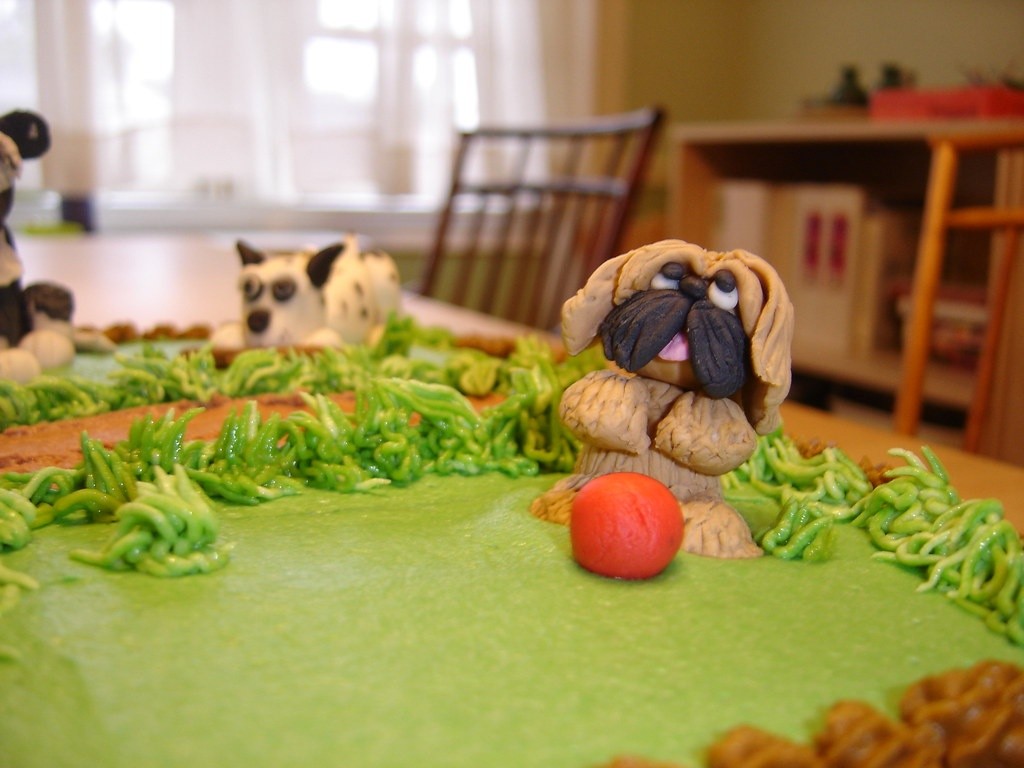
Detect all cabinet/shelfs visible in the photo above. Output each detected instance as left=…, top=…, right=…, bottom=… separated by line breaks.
left=555, top=109, right=1024, bottom=464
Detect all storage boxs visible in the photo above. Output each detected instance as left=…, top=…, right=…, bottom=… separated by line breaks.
left=772, top=179, right=871, bottom=350
left=700, top=176, right=763, bottom=260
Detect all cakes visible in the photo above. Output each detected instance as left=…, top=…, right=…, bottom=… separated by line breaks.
left=0, top=230, right=1024, bottom=768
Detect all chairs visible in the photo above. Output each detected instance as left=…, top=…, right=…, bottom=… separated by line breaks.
left=415, top=92, right=672, bottom=308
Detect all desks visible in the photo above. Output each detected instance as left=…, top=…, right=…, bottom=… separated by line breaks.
left=0, top=231, right=1024, bottom=768
